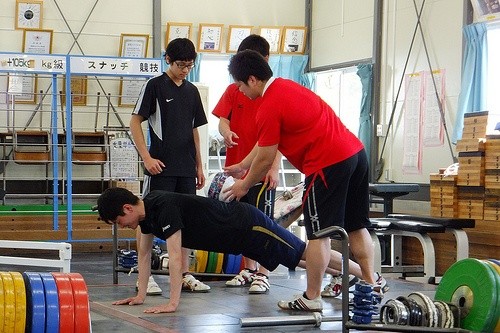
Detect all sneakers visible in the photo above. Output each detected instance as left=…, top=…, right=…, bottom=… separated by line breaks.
left=278, top=293, right=324, bottom=312
left=348, top=285, right=384, bottom=309
left=180, top=275, right=211, bottom=293
left=321, top=274, right=358, bottom=297
left=135, top=275, right=163, bottom=295
left=375, top=272, right=390, bottom=294
left=225, top=269, right=256, bottom=287
left=248, top=274, right=271, bottom=293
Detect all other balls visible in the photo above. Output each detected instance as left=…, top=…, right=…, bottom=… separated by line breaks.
left=203, top=172, right=236, bottom=203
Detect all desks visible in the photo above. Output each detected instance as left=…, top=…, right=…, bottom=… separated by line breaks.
left=0, top=133, right=48, bottom=204
left=58, top=135, right=112, bottom=205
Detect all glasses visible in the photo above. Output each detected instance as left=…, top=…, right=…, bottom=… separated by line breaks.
left=172, top=59, right=195, bottom=69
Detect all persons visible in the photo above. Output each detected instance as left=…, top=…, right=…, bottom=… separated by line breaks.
left=130, top=39, right=210, bottom=296
left=220, top=50, right=384, bottom=311
left=98, top=187, right=389, bottom=314
left=211, top=35, right=282, bottom=294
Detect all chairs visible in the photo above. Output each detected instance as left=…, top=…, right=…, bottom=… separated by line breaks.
left=59, top=92, right=110, bottom=165
left=6, top=90, right=52, bottom=165
